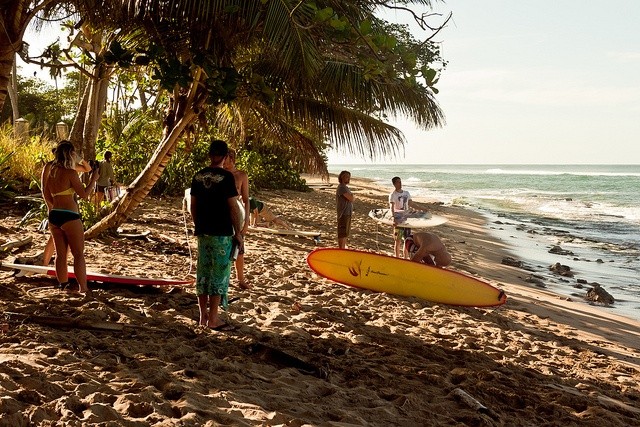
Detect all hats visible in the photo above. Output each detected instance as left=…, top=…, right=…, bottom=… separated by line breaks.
left=209, top=140, right=228, bottom=168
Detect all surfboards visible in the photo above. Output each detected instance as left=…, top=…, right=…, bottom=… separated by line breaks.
left=6, top=264, right=194, bottom=285
left=185, top=188, right=245, bottom=231
left=247, top=226, right=321, bottom=239
left=368, top=209, right=449, bottom=228
left=306, top=248, right=507, bottom=307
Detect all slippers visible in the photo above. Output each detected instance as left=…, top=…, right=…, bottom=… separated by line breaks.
left=211, top=320, right=235, bottom=331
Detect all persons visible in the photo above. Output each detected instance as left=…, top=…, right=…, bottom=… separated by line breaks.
left=40, top=139, right=93, bottom=266
left=389, top=176, right=413, bottom=258
left=238, top=194, right=292, bottom=230
left=336, top=170, right=353, bottom=248
left=405, top=232, right=451, bottom=266
left=222, top=147, right=252, bottom=288
left=43, top=142, right=101, bottom=293
left=96, top=150, right=120, bottom=212
left=190, top=139, right=244, bottom=332
